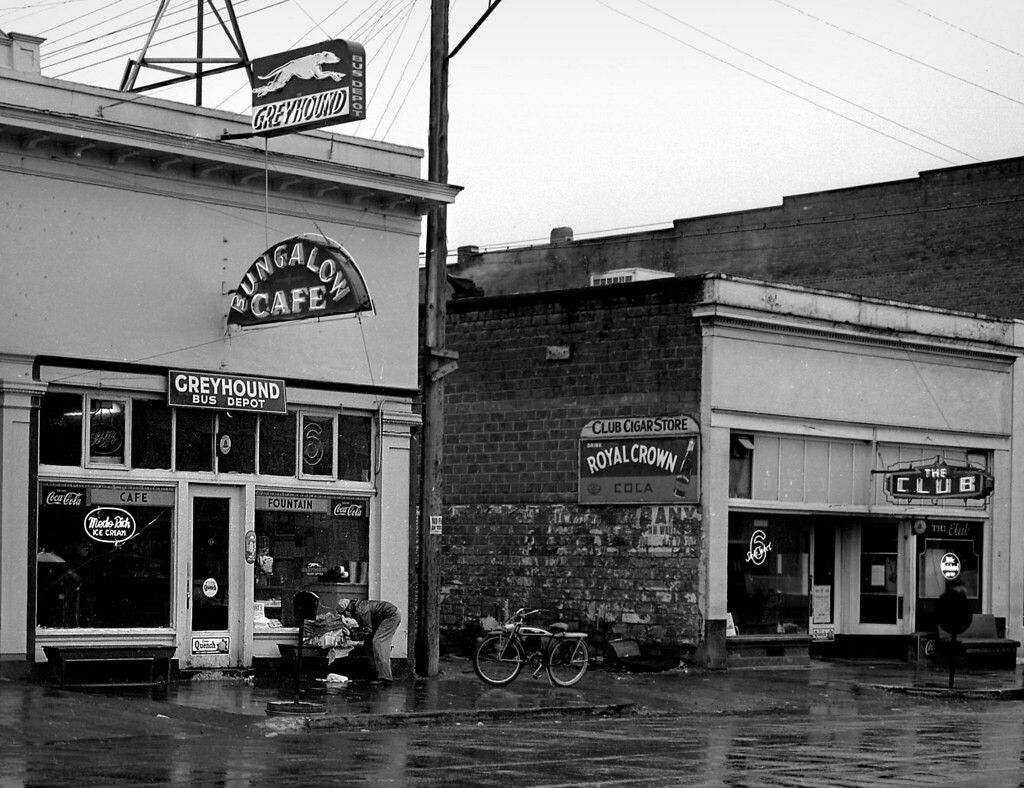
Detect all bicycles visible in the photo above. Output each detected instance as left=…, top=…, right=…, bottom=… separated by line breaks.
left=473, top=607, right=590, bottom=687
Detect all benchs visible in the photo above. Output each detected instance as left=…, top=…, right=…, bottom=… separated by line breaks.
left=935, top=613, right=1021, bottom=671
left=277, top=641, right=396, bottom=679
left=41, top=645, right=179, bottom=691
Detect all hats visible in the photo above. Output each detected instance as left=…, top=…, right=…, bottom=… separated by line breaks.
left=335, top=598, right=350, bottom=614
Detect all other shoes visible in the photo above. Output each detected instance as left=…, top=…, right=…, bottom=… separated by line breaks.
left=370, top=678, right=392, bottom=684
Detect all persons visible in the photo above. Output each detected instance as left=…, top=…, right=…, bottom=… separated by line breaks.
left=334, top=597, right=402, bottom=685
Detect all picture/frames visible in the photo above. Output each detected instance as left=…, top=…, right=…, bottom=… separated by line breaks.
left=274, top=511, right=297, bottom=537
